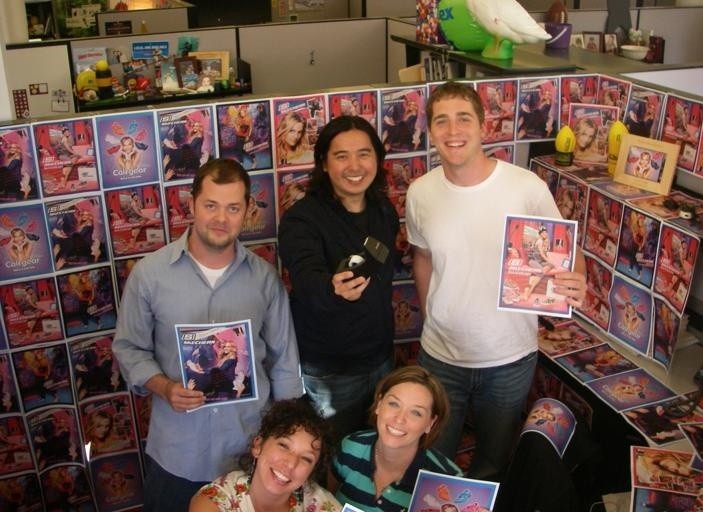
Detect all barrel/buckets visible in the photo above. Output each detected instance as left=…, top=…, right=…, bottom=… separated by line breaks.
left=545, top=22, right=573, bottom=50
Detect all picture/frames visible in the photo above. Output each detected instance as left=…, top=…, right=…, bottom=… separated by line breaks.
left=613, top=134, right=680, bottom=196
left=570, top=32, right=584, bottom=51
left=601, top=32, right=616, bottom=54
left=187, top=52, right=230, bottom=83
left=174, top=56, right=200, bottom=88
left=583, top=31, right=602, bottom=54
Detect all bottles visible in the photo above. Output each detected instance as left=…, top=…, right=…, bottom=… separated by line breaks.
left=93, top=61, right=114, bottom=97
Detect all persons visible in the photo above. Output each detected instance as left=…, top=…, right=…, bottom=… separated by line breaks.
left=0, top=82, right=703, bottom=512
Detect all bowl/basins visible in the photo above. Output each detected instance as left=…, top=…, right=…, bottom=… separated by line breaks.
left=620, top=45, right=649, bottom=60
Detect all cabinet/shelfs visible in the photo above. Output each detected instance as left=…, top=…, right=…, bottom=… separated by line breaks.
left=390, top=32, right=576, bottom=78
